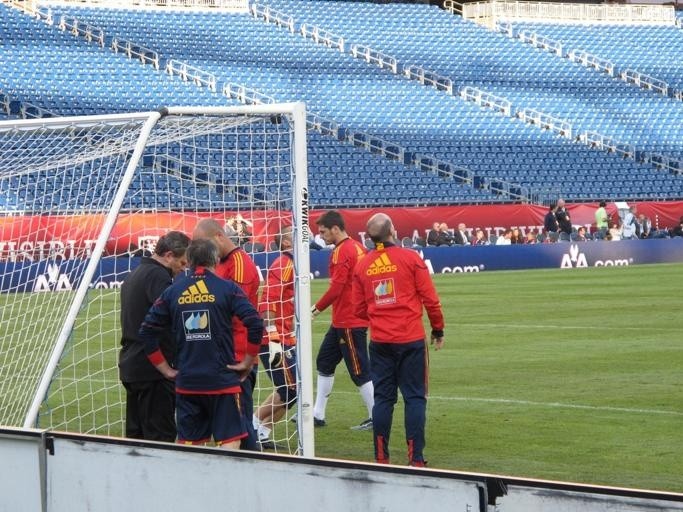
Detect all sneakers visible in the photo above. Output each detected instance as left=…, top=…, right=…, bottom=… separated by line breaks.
left=291, top=413, right=325, bottom=425
left=254, top=430, right=286, bottom=451
left=351, top=418, right=373, bottom=429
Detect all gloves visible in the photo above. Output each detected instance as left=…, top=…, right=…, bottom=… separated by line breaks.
left=267, top=332, right=283, bottom=368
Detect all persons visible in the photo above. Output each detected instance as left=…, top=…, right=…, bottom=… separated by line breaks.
left=136, top=240, right=151, bottom=256
left=194, top=218, right=262, bottom=453
left=118, top=232, right=190, bottom=442
left=427, top=198, right=683, bottom=246
left=138, top=239, right=264, bottom=451
left=252, top=223, right=296, bottom=450
left=124, top=244, right=135, bottom=257
left=351, top=212, right=445, bottom=467
left=223, top=213, right=253, bottom=245
left=311, top=211, right=374, bottom=431
